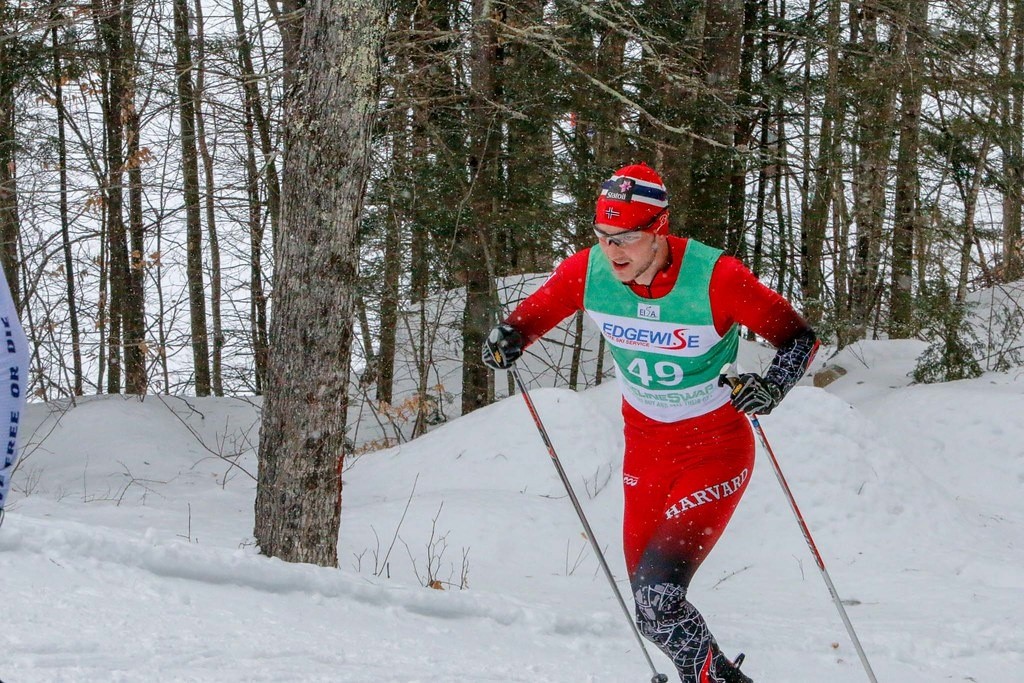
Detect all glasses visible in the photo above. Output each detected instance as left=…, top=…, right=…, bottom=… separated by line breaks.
left=589, top=205, right=671, bottom=247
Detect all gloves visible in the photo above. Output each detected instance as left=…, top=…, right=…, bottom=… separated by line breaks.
left=482, top=321, right=524, bottom=371
left=718, top=372, right=781, bottom=416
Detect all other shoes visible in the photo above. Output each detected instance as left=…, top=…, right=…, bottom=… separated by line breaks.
left=726, top=667, right=753, bottom=683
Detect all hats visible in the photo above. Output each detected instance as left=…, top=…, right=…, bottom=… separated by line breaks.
left=593, top=161, right=668, bottom=235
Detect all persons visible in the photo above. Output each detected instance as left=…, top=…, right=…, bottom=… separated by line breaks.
left=482, top=161, right=821, bottom=683
